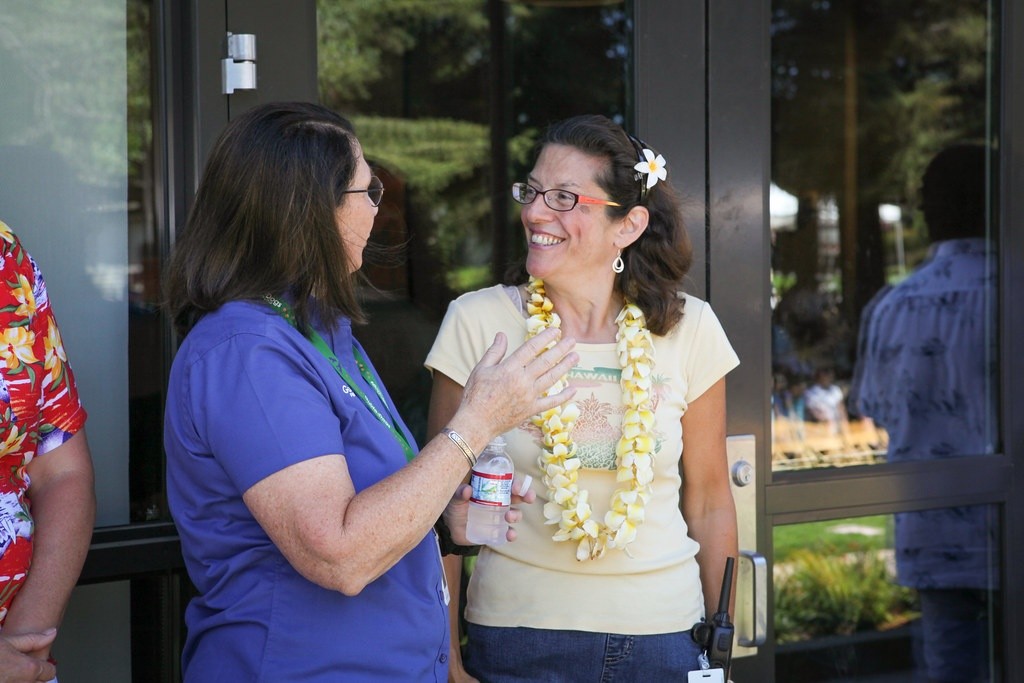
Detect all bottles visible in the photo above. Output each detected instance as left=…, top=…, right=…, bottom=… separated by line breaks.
left=465, top=435, right=515, bottom=546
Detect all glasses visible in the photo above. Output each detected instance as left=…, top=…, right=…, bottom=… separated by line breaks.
left=341, top=176, right=384, bottom=207
left=511, top=181, right=621, bottom=212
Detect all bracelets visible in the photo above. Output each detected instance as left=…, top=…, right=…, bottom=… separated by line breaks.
left=441, top=426, right=476, bottom=467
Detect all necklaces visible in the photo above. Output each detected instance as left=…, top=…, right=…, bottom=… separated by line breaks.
left=526, top=271, right=655, bottom=561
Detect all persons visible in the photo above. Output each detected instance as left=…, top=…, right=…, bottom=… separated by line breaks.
left=161, top=99, right=577, bottom=683
left=849, top=144, right=999, bottom=683
left=424, top=113, right=738, bottom=682
left=0, top=217, right=96, bottom=683
left=770, top=367, right=846, bottom=422
